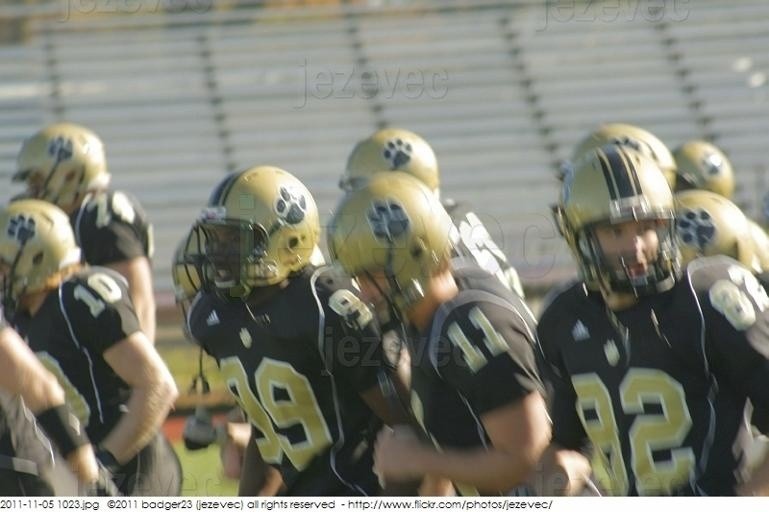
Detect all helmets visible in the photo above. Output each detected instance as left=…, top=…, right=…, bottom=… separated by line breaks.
left=183, top=165, right=320, bottom=295
left=10, top=122, right=111, bottom=214
left=0, top=199, right=82, bottom=313
left=343, top=127, right=441, bottom=199
left=547, top=123, right=769, bottom=297
left=325, top=172, right=459, bottom=279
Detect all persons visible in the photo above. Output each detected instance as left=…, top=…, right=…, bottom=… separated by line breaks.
left=0, top=121, right=768, bottom=496
